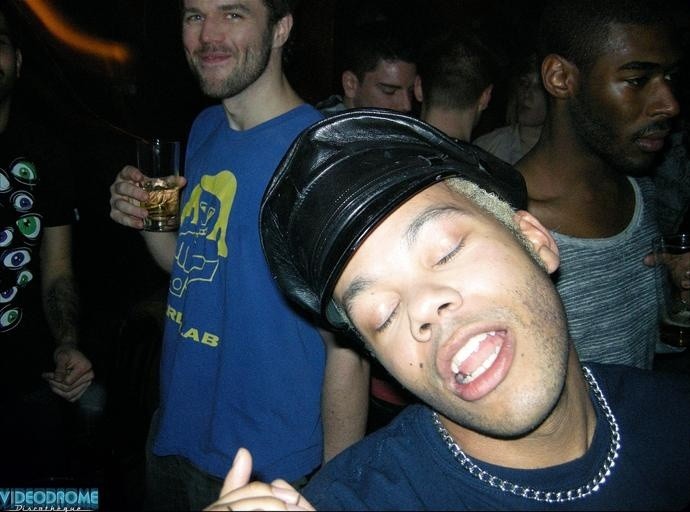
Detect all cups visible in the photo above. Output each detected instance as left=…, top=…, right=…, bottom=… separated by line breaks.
left=652, top=233, right=690, bottom=348
left=136, top=138, right=181, bottom=233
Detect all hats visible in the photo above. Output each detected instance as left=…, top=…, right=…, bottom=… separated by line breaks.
left=257, top=105, right=529, bottom=335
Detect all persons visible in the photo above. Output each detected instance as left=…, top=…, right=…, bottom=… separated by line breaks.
left=0, top=1, right=689, bottom=512
